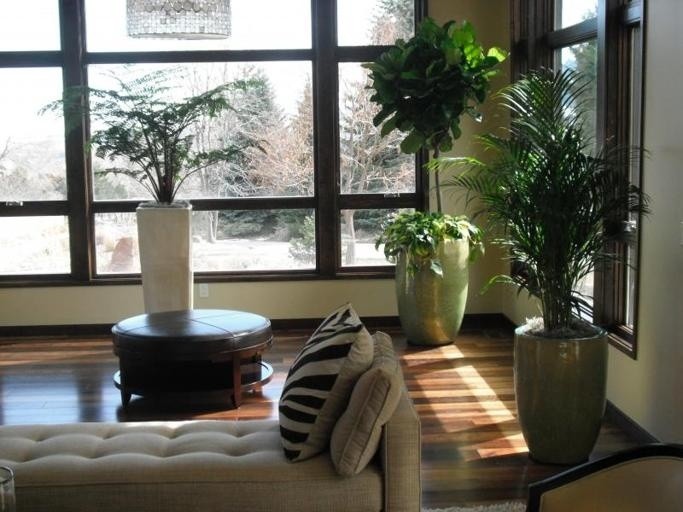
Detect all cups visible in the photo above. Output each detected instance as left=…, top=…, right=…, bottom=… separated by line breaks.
left=0, top=465, right=17, bottom=512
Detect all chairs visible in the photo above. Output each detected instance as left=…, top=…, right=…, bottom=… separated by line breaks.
left=520, top=441, right=683, bottom=512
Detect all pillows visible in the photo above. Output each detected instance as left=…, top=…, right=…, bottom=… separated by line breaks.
left=279, top=298, right=377, bottom=466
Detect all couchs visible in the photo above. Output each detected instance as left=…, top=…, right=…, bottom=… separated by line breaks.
left=329, top=325, right=402, bottom=475
left=0, top=298, right=422, bottom=512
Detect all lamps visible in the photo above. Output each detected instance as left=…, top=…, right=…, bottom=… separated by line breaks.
left=126, top=0, right=230, bottom=41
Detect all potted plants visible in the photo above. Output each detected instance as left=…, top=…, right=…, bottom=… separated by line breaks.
left=32, top=71, right=282, bottom=313
left=420, top=65, right=659, bottom=464
left=354, top=15, right=512, bottom=346
